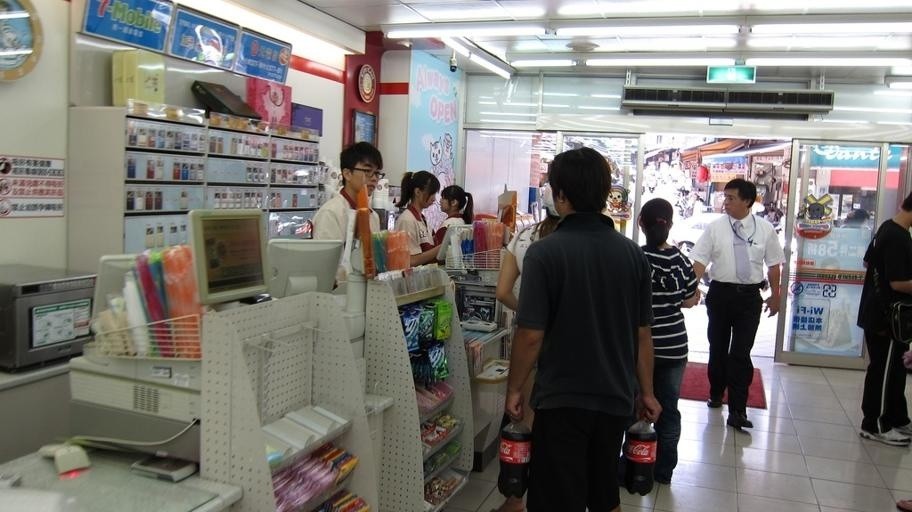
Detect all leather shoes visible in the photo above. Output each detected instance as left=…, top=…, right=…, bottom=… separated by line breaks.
left=708, top=396, right=723, bottom=408
left=728, top=414, right=752, bottom=429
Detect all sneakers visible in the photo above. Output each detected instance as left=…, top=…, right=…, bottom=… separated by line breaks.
left=859, top=427, right=911, bottom=446
left=895, top=420, right=911, bottom=435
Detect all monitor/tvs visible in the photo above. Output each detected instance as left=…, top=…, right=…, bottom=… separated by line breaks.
left=265, top=239, right=344, bottom=298
left=436, top=224, right=474, bottom=260
left=188, top=208, right=272, bottom=306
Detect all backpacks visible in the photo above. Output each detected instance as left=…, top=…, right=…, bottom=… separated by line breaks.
left=872, top=232, right=912, bottom=343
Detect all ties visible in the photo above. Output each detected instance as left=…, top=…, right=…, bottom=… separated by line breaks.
left=733, top=218, right=752, bottom=283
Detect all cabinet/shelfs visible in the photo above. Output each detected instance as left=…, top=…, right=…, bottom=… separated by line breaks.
left=460, top=322, right=509, bottom=472
left=67, top=98, right=319, bottom=272
left=0, top=360, right=84, bottom=465
left=200, top=291, right=395, bottom=512
left=360, top=267, right=474, bottom=512
left=0, top=448, right=243, bottom=511
left=95, top=315, right=198, bottom=364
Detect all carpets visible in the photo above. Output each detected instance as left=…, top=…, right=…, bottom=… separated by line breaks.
left=679, top=362, right=768, bottom=408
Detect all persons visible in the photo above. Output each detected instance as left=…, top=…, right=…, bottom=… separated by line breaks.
left=636, top=196, right=696, bottom=487
left=685, top=179, right=785, bottom=432
left=393, top=168, right=443, bottom=268
left=620, top=159, right=692, bottom=197
left=436, top=183, right=474, bottom=260
left=749, top=193, right=786, bottom=238
left=307, top=144, right=383, bottom=284
left=494, top=182, right=558, bottom=510
left=852, top=190, right=911, bottom=445
left=505, top=145, right=662, bottom=512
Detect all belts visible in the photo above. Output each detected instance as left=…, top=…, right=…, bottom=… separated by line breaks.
left=708, top=278, right=765, bottom=293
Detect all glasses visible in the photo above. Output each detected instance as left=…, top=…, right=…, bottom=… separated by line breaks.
left=350, top=166, right=386, bottom=179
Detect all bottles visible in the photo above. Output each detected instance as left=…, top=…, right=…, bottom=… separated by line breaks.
left=625, top=408, right=657, bottom=495
left=498, top=412, right=533, bottom=500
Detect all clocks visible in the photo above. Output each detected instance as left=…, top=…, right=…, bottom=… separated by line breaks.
left=357, top=63, right=377, bottom=102
left=0, top=0, right=43, bottom=81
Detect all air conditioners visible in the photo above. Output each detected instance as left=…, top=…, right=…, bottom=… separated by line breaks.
left=620, top=69, right=835, bottom=113
